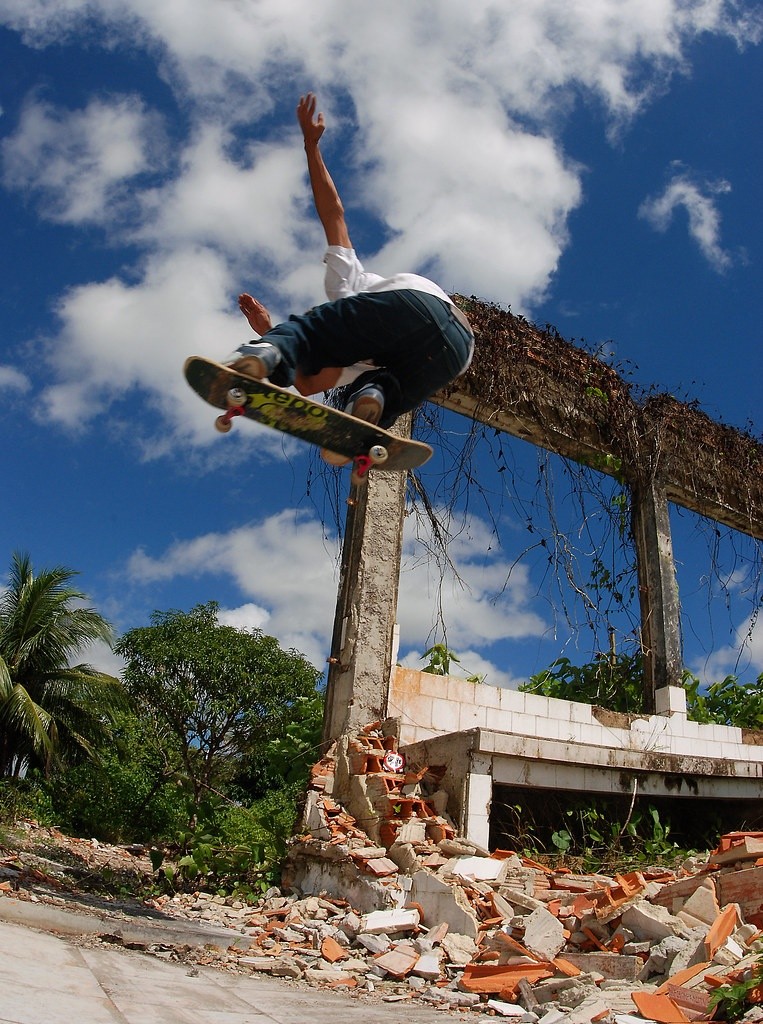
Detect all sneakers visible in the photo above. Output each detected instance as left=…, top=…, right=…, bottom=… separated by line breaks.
left=222, top=342, right=282, bottom=380
left=322, top=383, right=384, bottom=466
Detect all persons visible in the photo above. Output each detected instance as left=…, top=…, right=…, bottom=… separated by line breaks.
left=221, top=92, right=476, bottom=467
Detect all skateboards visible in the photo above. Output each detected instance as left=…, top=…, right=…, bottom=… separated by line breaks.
left=183, top=355, right=434, bottom=486
